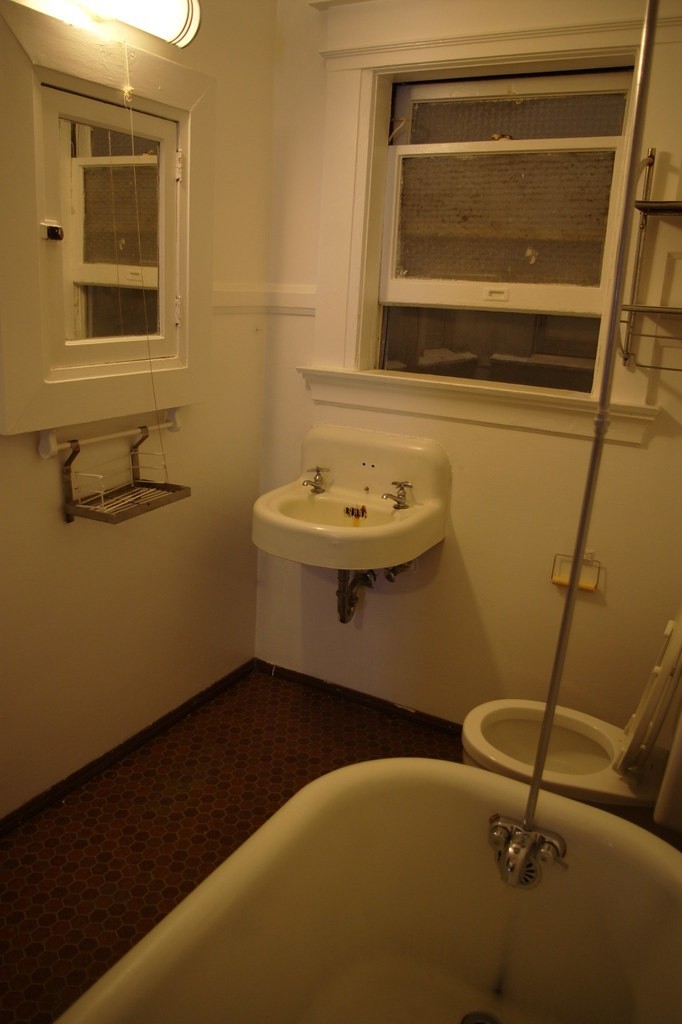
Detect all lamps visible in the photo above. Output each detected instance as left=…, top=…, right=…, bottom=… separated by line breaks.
left=8, top=0, right=203, bottom=50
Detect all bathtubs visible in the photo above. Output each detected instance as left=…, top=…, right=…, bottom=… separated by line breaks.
left=45, top=759, right=680, bottom=1024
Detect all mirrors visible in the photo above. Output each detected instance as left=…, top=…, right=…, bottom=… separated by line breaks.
left=0, top=1, right=218, bottom=427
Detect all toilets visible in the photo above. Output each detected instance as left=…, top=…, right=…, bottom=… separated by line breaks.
left=461, top=617, right=682, bottom=812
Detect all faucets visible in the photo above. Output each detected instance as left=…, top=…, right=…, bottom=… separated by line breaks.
left=302, top=467, right=330, bottom=494
left=381, top=482, right=414, bottom=509
left=502, top=831, right=536, bottom=886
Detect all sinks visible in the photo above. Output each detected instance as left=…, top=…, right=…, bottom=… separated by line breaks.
left=252, top=424, right=451, bottom=569
